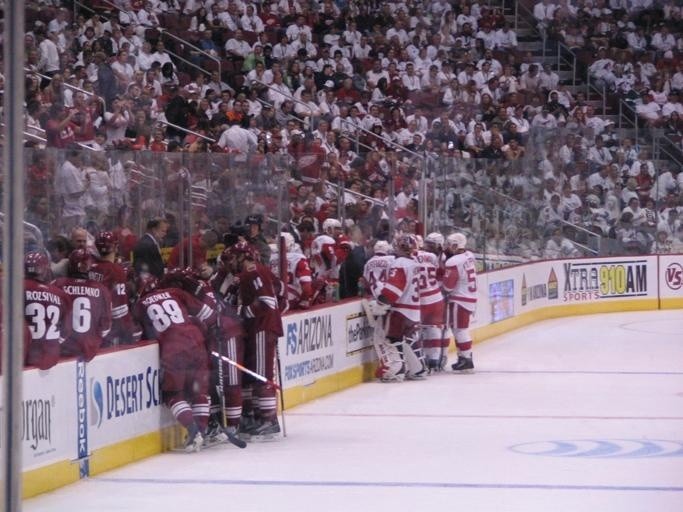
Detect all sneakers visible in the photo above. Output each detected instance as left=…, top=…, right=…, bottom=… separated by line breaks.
left=247, top=417, right=281, bottom=435
left=241, top=417, right=263, bottom=435
left=452, top=356, right=463, bottom=368
left=454, top=357, right=474, bottom=370
left=183, top=425, right=200, bottom=448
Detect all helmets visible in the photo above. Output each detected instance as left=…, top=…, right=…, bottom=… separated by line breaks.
left=231, top=240, right=256, bottom=259
left=251, top=243, right=271, bottom=258
left=25, top=250, right=48, bottom=274
left=135, top=272, right=160, bottom=294
left=221, top=248, right=236, bottom=259
left=95, top=231, right=119, bottom=251
left=446, top=232, right=467, bottom=249
left=164, top=268, right=188, bottom=286
left=374, top=241, right=389, bottom=255
left=323, top=218, right=341, bottom=235
left=277, top=232, right=294, bottom=250
left=393, top=235, right=417, bottom=250
left=425, top=233, right=444, bottom=249
left=69, top=249, right=91, bottom=272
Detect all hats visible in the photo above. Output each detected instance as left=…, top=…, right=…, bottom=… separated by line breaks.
left=604, top=119, right=615, bottom=126
left=433, top=122, right=441, bottom=126
left=442, top=59, right=451, bottom=66
left=324, top=80, right=335, bottom=87
left=466, top=62, right=475, bottom=67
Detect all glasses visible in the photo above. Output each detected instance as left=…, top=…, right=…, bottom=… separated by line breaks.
left=433, top=126, right=441, bottom=129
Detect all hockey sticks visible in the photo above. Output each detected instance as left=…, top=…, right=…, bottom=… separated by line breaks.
left=210, top=351, right=280, bottom=391
left=216, top=316, right=247, bottom=449
left=275, top=340, right=287, bottom=437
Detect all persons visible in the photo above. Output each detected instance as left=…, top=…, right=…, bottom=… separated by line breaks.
left=155, top=264, right=244, bottom=443
left=229, top=240, right=284, bottom=442
left=22, top=249, right=69, bottom=371
left=129, top=272, right=217, bottom=452
left=376, top=234, right=427, bottom=383
left=422, top=231, right=451, bottom=371
left=54, top=247, right=113, bottom=362
left=0, top=2, right=682, bottom=309
left=439, top=232, right=476, bottom=374
left=87, top=230, right=142, bottom=348
left=414, top=249, right=444, bottom=375
